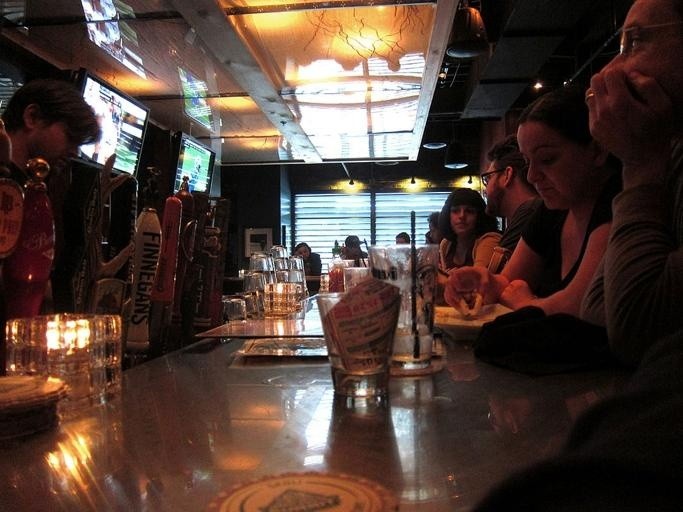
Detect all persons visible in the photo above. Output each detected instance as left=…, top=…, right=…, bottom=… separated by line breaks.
left=431, top=185, right=506, bottom=286
left=423, top=209, right=448, bottom=244
left=394, top=233, right=411, bottom=245
left=573, top=0, right=682, bottom=363
left=340, top=236, right=369, bottom=260
left=444, top=136, right=544, bottom=274
left=484, top=391, right=540, bottom=447
left=2, top=78, right=103, bottom=315
left=443, top=89, right=625, bottom=322
left=469, top=330, right=682, bottom=511
left=292, top=240, right=323, bottom=276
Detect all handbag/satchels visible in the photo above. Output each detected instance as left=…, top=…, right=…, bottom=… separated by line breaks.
left=472, top=306, right=609, bottom=379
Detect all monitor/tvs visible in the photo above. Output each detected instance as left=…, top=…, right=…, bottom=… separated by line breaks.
left=61, top=68, right=151, bottom=180
left=81, top=1, right=150, bottom=82
left=165, top=128, right=216, bottom=203
left=178, top=66, right=216, bottom=131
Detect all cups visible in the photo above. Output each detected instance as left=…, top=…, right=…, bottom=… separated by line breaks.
left=340, top=258, right=371, bottom=293
left=314, top=292, right=403, bottom=376
left=2, top=312, right=122, bottom=376
left=364, top=242, right=440, bottom=371
left=219, top=245, right=307, bottom=325
left=55, top=364, right=123, bottom=420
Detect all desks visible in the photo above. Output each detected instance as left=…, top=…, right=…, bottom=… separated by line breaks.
left=0, top=288, right=683, bottom=512
left=223, top=275, right=322, bottom=282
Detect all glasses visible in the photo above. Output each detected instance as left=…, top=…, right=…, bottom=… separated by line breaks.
left=480, top=167, right=515, bottom=187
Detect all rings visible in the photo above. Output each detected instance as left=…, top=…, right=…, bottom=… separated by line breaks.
left=584, top=93, right=596, bottom=102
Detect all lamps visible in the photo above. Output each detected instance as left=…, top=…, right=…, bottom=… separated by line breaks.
left=444, top=139, right=471, bottom=170
left=447, top=0, right=489, bottom=58
left=339, top=162, right=356, bottom=190
left=430, top=87, right=465, bottom=117
left=463, top=161, right=475, bottom=190
left=407, top=161, right=420, bottom=190
left=422, top=117, right=450, bottom=150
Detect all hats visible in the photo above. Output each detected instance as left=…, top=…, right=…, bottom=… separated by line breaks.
left=345, top=236, right=362, bottom=246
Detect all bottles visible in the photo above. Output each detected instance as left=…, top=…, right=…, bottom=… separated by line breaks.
left=326, top=239, right=346, bottom=292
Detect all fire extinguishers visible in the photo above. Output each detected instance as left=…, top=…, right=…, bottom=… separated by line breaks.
left=175, top=175, right=196, bottom=273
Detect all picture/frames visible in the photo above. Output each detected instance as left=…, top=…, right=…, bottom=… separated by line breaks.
left=244, top=228, right=274, bottom=257
